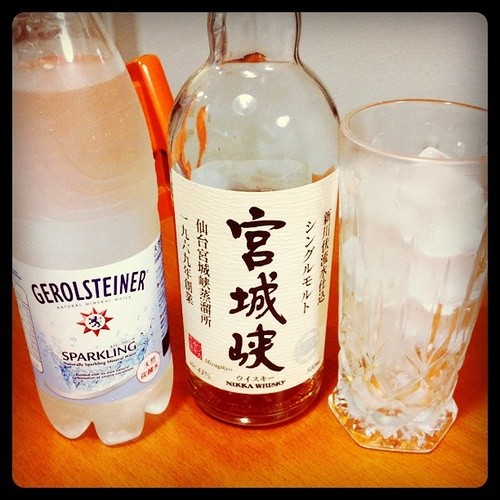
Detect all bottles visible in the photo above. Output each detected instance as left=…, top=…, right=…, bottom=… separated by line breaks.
left=167, top=8, right=345, bottom=430
left=9, top=10, right=173, bottom=447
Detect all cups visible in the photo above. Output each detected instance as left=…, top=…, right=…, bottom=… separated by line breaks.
left=327, top=95, right=490, bottom=455
